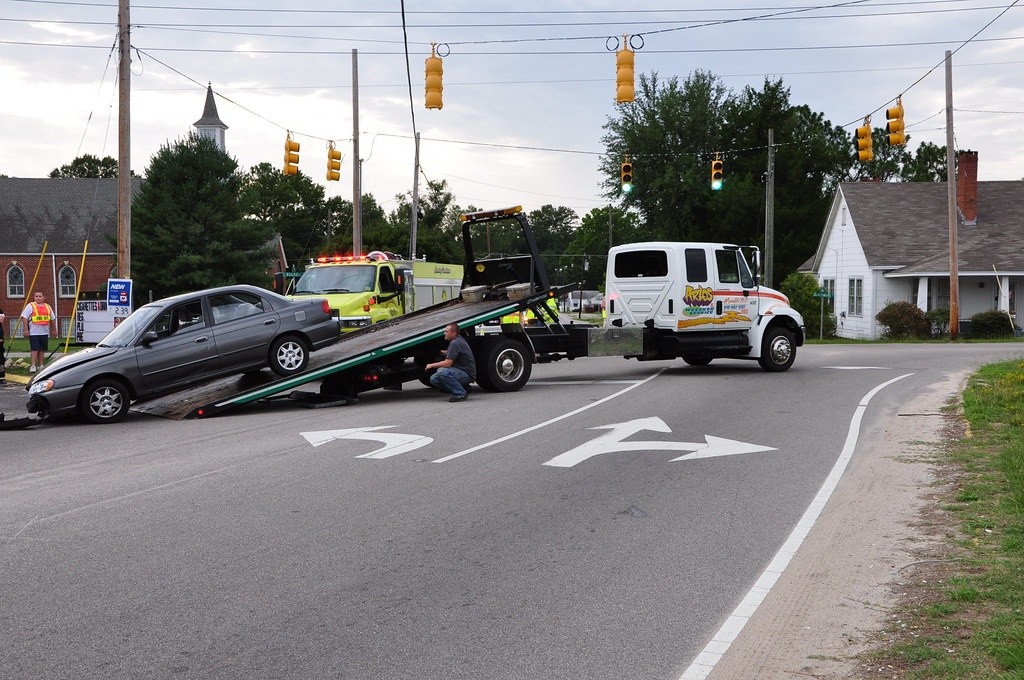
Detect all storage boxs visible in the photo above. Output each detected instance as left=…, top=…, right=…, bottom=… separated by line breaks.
left=461, top=285, right=487, bottom=303
left=506, top=282, right=536, bottom=300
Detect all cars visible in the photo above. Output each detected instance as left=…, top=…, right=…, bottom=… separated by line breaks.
left=25, top=284, right=342, bottom=425
left=558, top=288, right=605, bottom=314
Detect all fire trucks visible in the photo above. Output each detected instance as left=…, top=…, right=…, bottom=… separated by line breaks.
left=280, top=251, right=483, bottom=336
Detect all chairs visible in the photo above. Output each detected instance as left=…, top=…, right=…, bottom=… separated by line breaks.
left=177, top=309, right=194, bottom=330
left=212, top=307, right=220, bottom=324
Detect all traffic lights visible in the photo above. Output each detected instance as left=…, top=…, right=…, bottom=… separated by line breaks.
left=620, top=163, right=633, bottom=193
left=326, top=147, right=341, bottom=181
left=854, top=123, right=873, bottom=162
left=886, top=101, right=905, bottom=146
left=283, top=138, right=299, bottom=176
left=712, top=161, right=725, bottom=190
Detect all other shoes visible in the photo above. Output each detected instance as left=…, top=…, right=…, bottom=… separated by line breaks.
left=39, top=365, right=44, bottom=371
left=29, top=365, right=36, bottom=372
left=0, top=379, right=7, bottom=384
left=449, top=393, right=468, bottom=402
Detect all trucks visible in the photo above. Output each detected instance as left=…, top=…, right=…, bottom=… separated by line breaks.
left=317, top=240, right=806, bottom=393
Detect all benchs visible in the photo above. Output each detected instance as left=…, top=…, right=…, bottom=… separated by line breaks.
left=235, top=302, right=263, bottom=317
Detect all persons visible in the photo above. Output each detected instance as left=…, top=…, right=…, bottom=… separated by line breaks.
left=20, top=290, right=57, bottom=373
left=0, top=309, right=7, bottom=384
left=500, top=297, right=559, bottom=328
left=425, top=323, right=477, bottom=402
